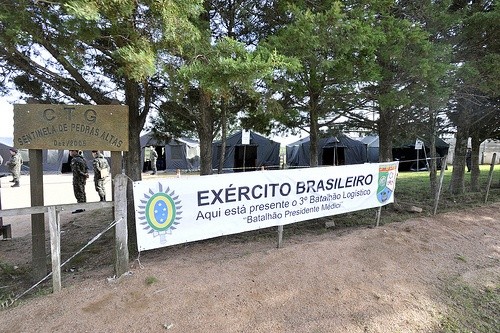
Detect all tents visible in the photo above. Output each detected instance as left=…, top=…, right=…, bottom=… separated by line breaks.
left=212, top=127, right=281, bottom=172
left=0, top=143, right=107, bottom=174
left=139, top=127, right=187, bottom=175
left=286, top=126, right=450, bottom=171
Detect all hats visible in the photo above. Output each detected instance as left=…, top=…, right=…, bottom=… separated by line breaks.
left=9, top=147, right=18, bottom=152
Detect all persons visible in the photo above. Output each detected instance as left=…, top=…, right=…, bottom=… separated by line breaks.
left=5, top=147, right=24, bottom=188
left=465, top=148, right=473, bottom=172
left=150, top=145, right=158, bottom=176
left=92, top=150, right=110, bottom=202
left=70, top=149, right=89, bottom=214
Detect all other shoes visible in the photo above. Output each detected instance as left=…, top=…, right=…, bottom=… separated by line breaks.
left=72, top=209, right=85, bottom=213
left=150, top=173, right=156, bottom=175
left=11, top=183, right=19, bottom=187
left=9, top=179, right=14, bottom=182
